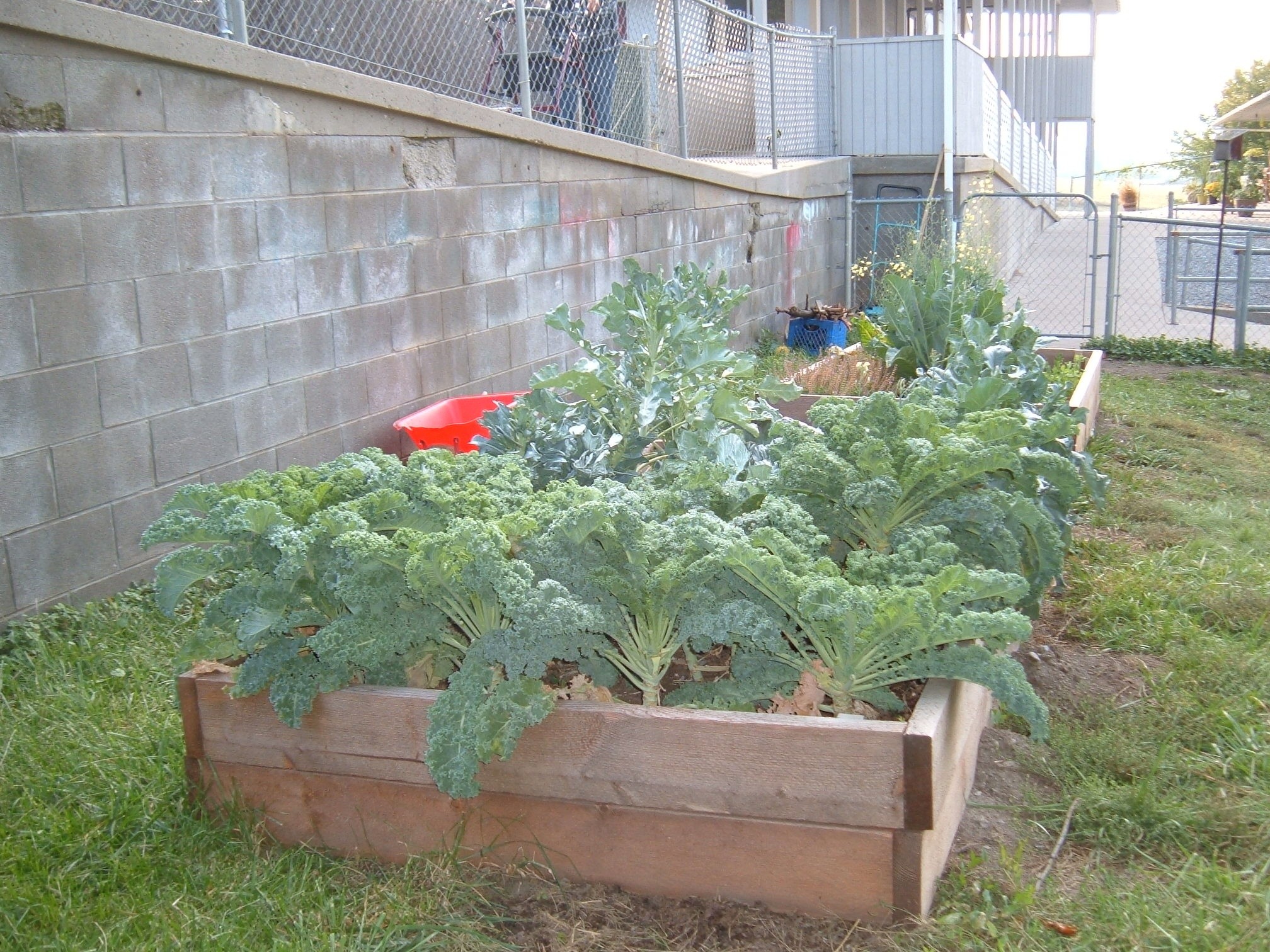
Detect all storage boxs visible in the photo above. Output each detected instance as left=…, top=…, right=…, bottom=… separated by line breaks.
left=787, top=315, right=848, bottom=360
left=392, top=391, right=530, bottom=454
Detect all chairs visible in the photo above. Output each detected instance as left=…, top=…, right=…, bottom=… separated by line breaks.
left=482, top=7, right=605, bottom=135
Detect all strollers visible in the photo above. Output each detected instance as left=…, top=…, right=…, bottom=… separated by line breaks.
left=472, top=0, right=607, bottom=138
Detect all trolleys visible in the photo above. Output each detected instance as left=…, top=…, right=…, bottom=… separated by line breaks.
left=392, top=391, right=534, bottom=454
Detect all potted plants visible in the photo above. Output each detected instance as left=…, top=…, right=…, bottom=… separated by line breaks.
left=1121, top=183, right=1137, bottom=212
left=1184, top=150, right=1270, bottom=217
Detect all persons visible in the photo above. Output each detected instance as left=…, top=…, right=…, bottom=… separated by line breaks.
left=512, top=0, right=618, bottom=137
